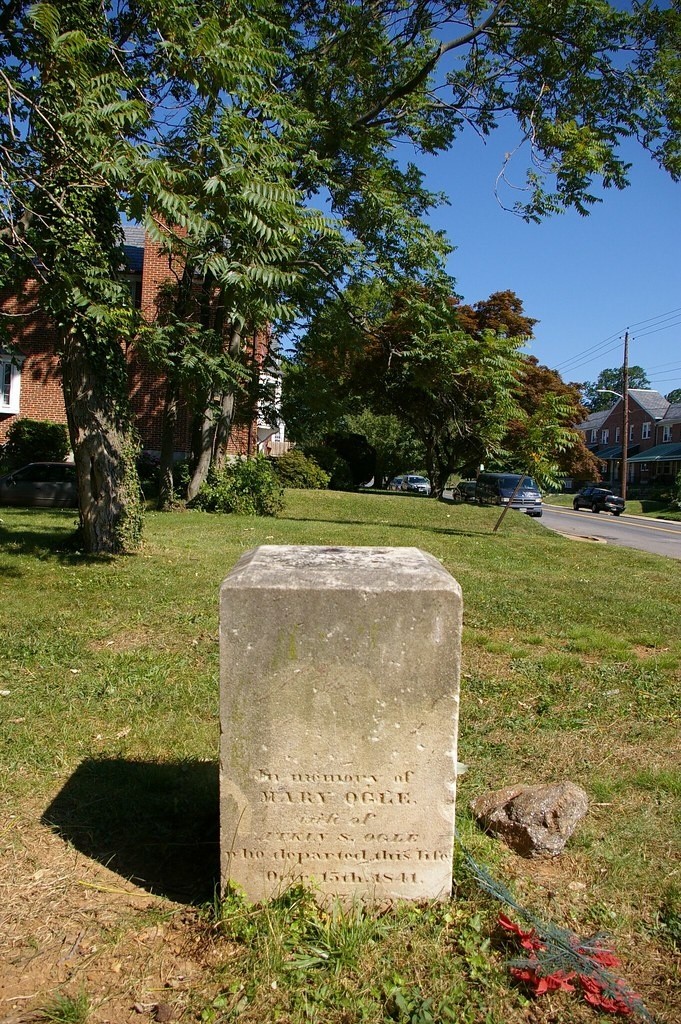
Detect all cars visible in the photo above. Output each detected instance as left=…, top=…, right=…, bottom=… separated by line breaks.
left=402, top=475, right=432, bottom=495
left=0, top=461, right=78, bottom=509
left=389, top=477, right=403, bottom=491
left=452, top=481, right=477, bottom=503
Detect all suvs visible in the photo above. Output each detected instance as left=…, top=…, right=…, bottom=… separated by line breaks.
left=573, top=486, right=626, bottom=517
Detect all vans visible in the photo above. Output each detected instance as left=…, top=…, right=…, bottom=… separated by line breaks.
left=475, top=473, right=543, bottom=517
